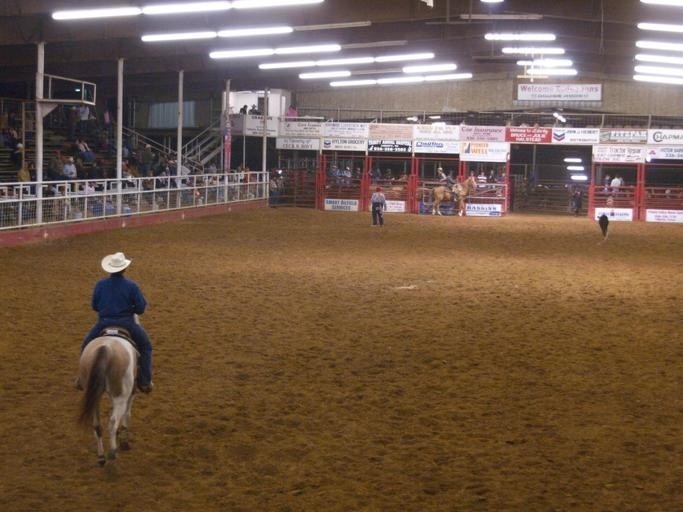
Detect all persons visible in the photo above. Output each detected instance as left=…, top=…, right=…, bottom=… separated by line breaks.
left=75, top=249, right=154, bottom=397
left=522, top=169, right=537, bottom=196
left=599, top=170, right=682, bottom=207
left=529, top=182, right=589, bottom=216
left=327, top=162, right=506, bottom=202
left=0, top=103, right=287, bottom=231
left=369, top=186, right=388, bottom=227
left=284, top=102, right=300, bottom=121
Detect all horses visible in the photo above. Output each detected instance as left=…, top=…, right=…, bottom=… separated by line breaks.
left=71, top=312, right=142, bottom=466
left=426, top=174, right=477, bottom=217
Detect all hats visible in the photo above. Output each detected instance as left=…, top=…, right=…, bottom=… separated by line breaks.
left=100, top=253, right=134, bottom=275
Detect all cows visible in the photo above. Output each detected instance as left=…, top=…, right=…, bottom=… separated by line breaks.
left=597, top=214, right=609, bottom=237
